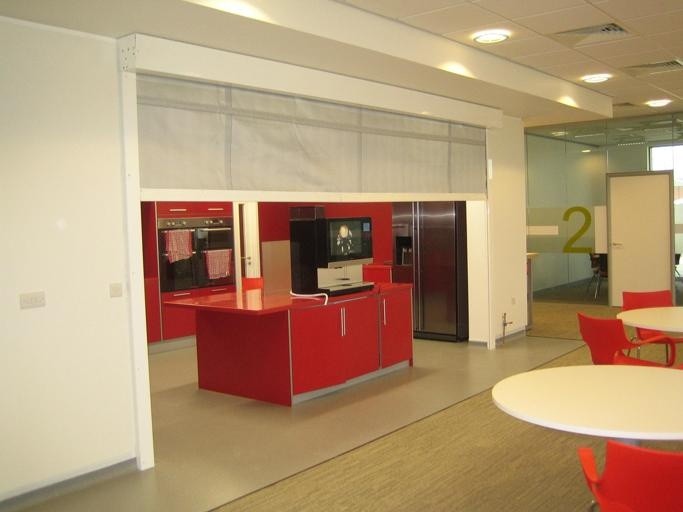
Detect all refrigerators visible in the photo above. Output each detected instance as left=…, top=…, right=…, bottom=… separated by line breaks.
left=389, top=201, right=469, bottom=342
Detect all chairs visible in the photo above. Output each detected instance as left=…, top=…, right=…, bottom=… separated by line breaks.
left=576, top=440, right=683, bottom=511
left=585, top=253, right=607, bottom=302
left=576, top=291, right=683, bottom=369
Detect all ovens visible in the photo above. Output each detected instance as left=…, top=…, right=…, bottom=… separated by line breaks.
left=158, top=218, right=232, bottom=290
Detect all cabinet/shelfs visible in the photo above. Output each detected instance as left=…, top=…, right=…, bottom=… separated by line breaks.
left=196, top=296, right=380, bottom=407
left=161, top=285, right=235, bottom=351
left=156, top=202, right=232, bottom=218
left=380, top=288, right=412, bottom=377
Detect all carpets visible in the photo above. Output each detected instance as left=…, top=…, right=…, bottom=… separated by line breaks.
left=2, top=330, right=585, bottom=511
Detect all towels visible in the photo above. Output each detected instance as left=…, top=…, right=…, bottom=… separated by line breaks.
left=205, top=249, right=232, bottom=279
left=165, top=230, right=192, bottom=264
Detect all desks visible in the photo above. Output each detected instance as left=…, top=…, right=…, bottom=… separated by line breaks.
left=491, top=364, right=682, bottom=449
